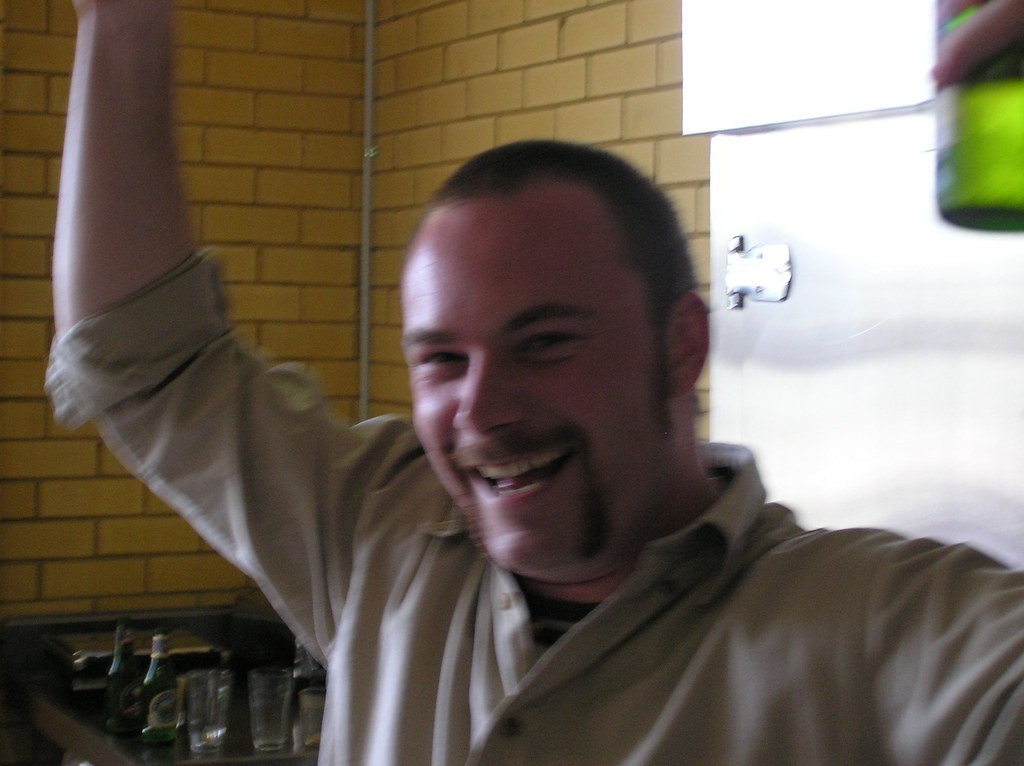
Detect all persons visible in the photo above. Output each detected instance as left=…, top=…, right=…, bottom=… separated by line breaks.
left=47, top=0, right=1024, bottom=766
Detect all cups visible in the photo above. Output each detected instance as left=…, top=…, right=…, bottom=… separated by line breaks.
left=299, top=687, right=327, bottom=752
left=188, top=670, right=233, bottom=754
left=248, top=667, right=293, bottom=752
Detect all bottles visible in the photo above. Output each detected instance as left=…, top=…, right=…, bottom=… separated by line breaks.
left=140, top=628, right=179, bottom=746
left=106, top=623, right=140, bottom=738
left=933, top=0, right=1024, bottom=231
